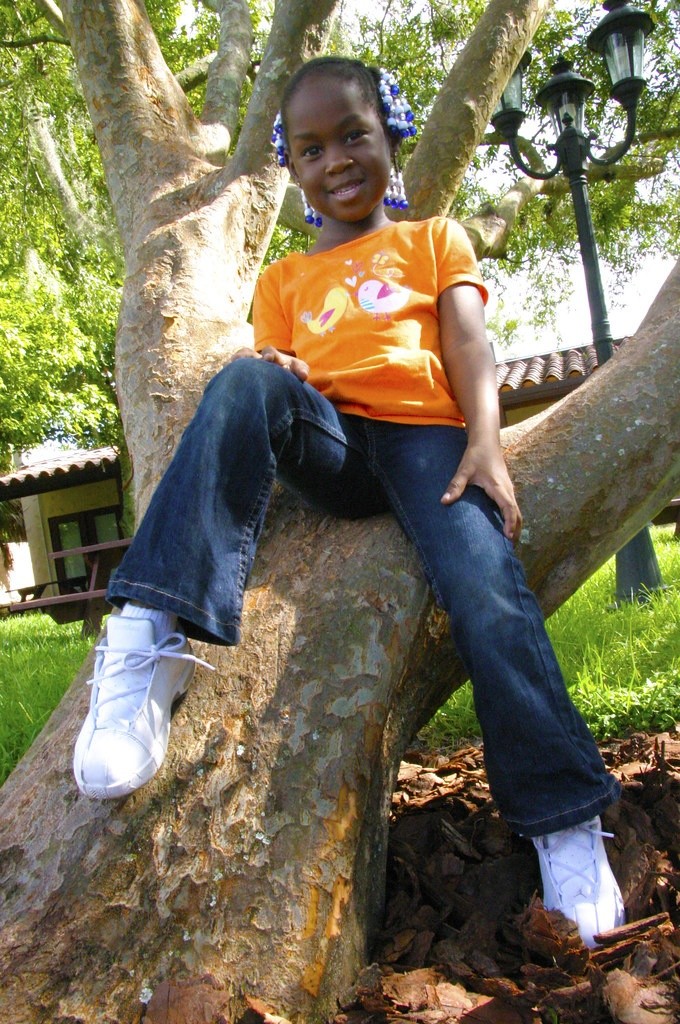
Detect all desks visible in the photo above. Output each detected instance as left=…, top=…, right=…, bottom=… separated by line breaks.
left=7, top=575, right=88, bottom=602
left=48, top=537, right=134, bottom=640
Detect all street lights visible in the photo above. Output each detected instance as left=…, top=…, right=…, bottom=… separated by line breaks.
left=488, top=1, right=662, bottom=602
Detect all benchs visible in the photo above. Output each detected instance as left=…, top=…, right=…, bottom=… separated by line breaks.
left=8, top=589, right=106, bottom=612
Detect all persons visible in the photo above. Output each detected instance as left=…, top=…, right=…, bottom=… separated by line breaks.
left=74, top=56, right=626, bottom=949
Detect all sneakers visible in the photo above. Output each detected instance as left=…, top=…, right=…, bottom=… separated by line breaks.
left=72, top=614, right=216, bottom=799
left=530, top=814, right=625, bottom=951
